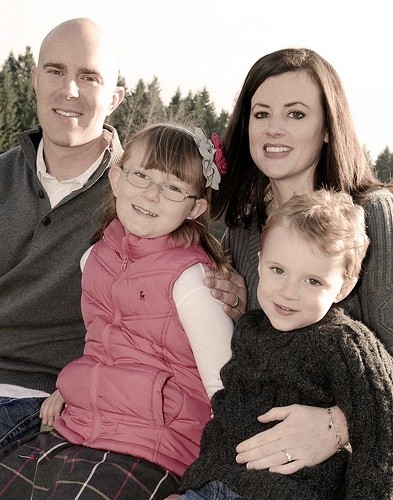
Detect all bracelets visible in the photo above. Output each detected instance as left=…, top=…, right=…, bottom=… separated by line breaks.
left=325, top=405, right=343, bottom=454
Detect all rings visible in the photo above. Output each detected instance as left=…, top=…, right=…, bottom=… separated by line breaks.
left=281, top=449, right=293, bottom=461
left=231, top=295, right=240, bottom=308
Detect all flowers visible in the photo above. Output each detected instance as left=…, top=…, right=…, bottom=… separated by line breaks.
left=194, top=127, right=226, bottom=189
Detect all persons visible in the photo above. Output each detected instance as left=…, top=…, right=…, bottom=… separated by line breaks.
left=0, top=18, right=247, bottom=453
left=207, top=47, right=393, bottom=475
left=0, top=123, right=238, bottom=500
left=157, top=188, right=392, bottom=499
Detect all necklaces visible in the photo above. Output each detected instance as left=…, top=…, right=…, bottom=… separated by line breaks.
left=271, top=198, right=276, bottom=215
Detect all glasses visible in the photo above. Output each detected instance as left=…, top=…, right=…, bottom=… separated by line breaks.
left=120, top=166, right=199, bottom=202
